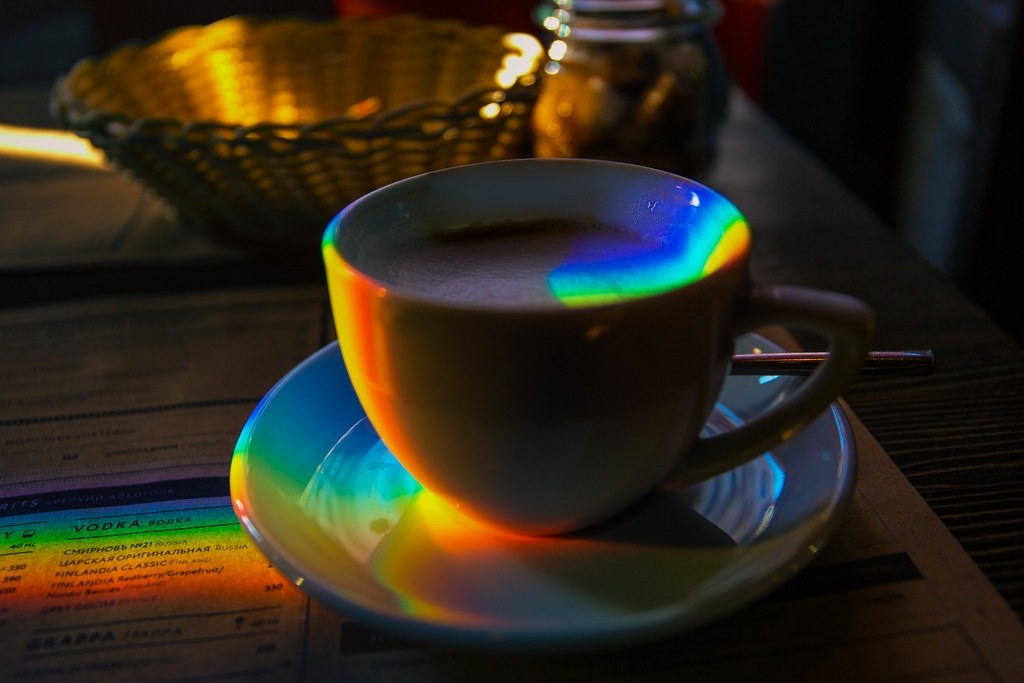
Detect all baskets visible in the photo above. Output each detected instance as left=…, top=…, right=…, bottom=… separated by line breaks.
left=53, top=10, right=545, bottom=246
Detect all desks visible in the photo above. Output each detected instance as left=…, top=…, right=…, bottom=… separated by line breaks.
left=0, top=0, right=1024, bottom=683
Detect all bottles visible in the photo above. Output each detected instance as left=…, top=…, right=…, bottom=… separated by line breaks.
left=534, top=0, right=727, bottom=181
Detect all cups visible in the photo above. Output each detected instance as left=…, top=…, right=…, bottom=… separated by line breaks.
left=323, top=159, right=872, bottom=535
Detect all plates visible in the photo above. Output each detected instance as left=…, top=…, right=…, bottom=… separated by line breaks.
left=230, top=334, right=858, bottom=644
